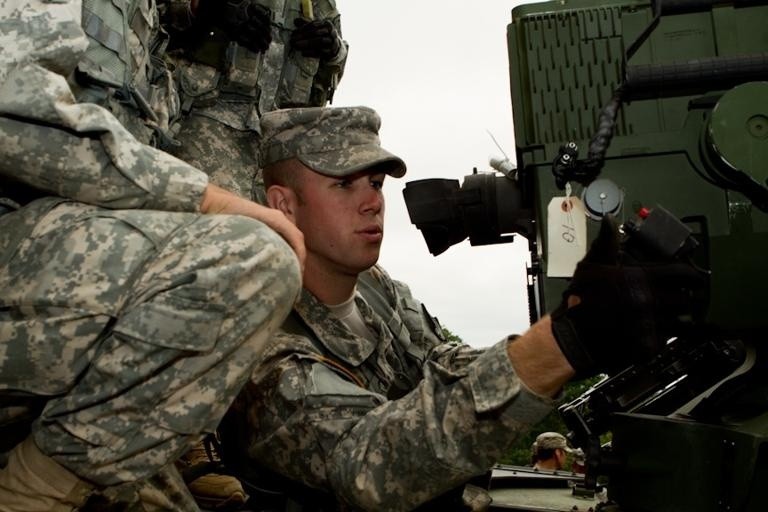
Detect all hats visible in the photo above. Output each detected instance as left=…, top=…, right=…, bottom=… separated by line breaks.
left=532, top=431, right=573, bottom=456
left=257, top=104, right=407, bottom=178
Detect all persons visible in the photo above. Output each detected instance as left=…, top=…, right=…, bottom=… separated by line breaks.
left=0, top=0, right=309, bottom=512
left=219, top=104, right=716, bottom=511
left=169, top=0, right=350, bottom=507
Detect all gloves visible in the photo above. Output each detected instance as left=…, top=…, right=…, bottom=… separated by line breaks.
left=292, top=17, right=341, bottom=60
left=196, top=0, right=273, bottom=55
left=548, top=210, right=713, bottom=377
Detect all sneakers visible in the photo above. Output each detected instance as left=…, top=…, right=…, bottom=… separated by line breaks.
left=174, top=439, right=252, bottom=512
